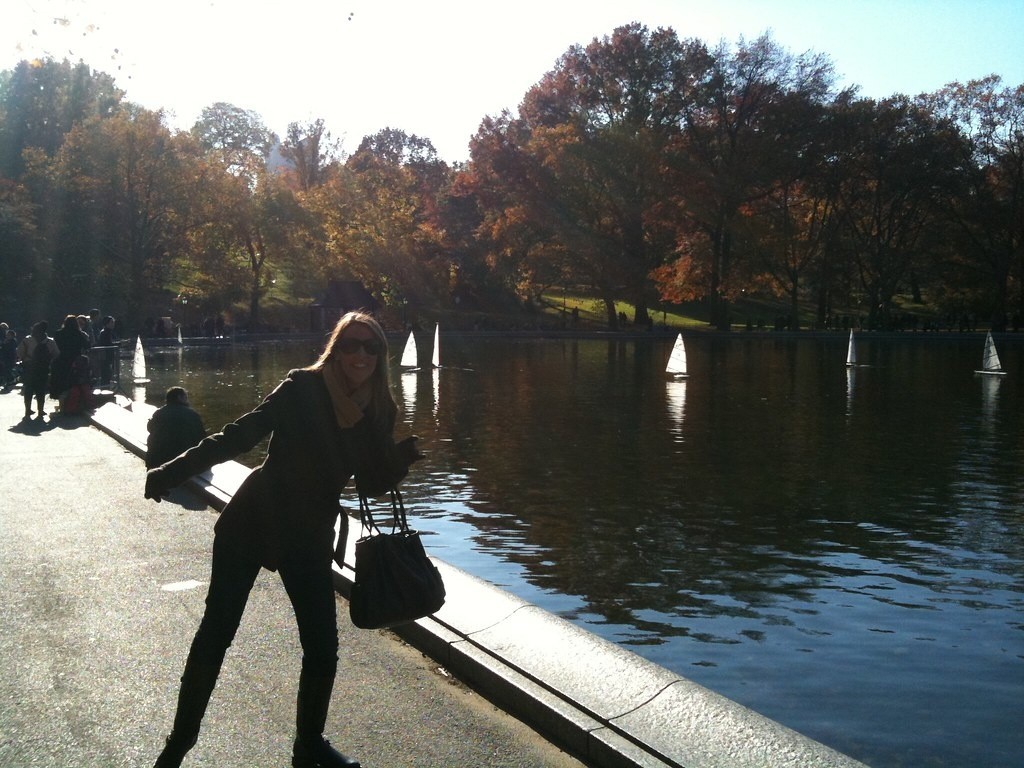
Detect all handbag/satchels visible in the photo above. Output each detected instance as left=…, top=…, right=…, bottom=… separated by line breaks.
left=349, top=480, right=446, bottom=629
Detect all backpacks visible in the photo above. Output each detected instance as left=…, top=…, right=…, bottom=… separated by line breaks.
left=32, top=338, right=50, bottom=378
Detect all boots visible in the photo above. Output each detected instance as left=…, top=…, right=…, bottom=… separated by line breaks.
left=154, top=656, right=219, bottom=768
left=291, top=669, right=360, bottom=768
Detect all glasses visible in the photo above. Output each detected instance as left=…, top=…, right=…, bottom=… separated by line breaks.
left=337, top=338, right=380, bottom=355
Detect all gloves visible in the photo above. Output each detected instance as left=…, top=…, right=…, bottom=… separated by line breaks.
left=394, top=434, right=425, bottom=466
left=144, top=466, right=181, bottom=503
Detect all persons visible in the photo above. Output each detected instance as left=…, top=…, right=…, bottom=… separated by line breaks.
left=145, top=310, right=426, bottom=768
left=202, top=315, right=225, bottom=337
left=619, top=311, right=627, bottom=321
left=146, top=386, right=207, bottom=472
left=0, top=309, right=131, bottom=415
left=572, top=306, right=578, bottom=319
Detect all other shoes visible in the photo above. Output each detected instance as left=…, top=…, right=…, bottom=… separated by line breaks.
left=39, top=411, right=46, bottom=416
left=26, top=410, right=34, bottom=415
